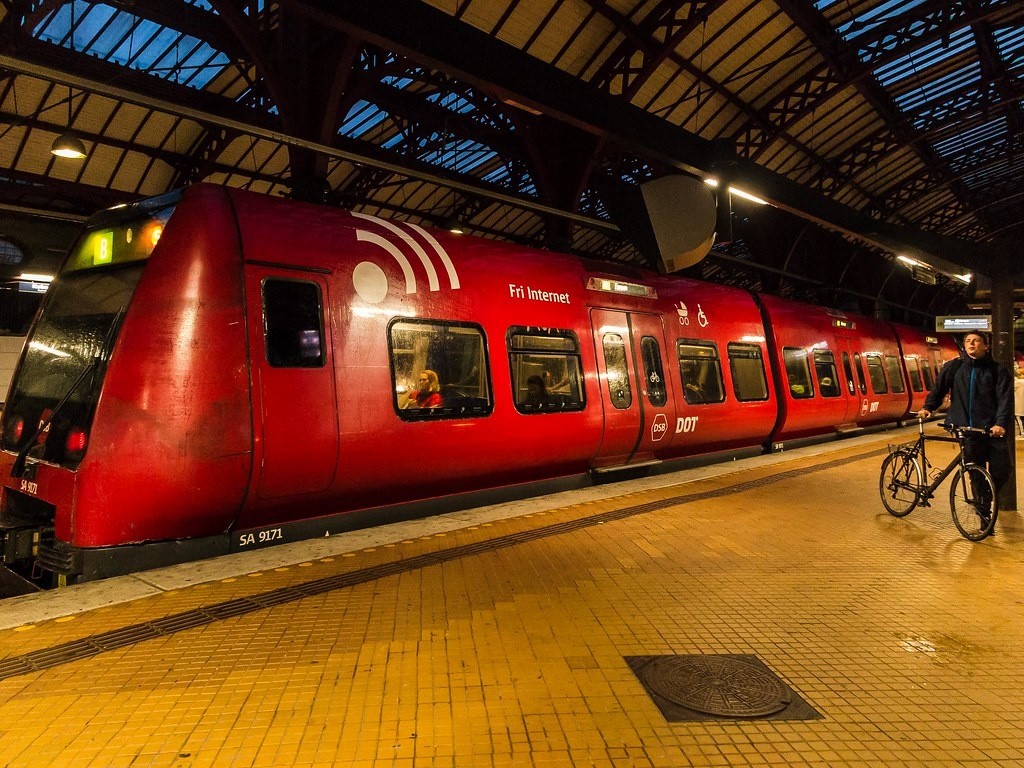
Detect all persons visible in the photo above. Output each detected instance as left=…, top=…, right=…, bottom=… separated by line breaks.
left=407, top=370, right=442, bottom=408
left=1014, top=356, right=1024, bottom=440
left=918, top=329, right=1012, bottom=535
left=788, top=374, right=806, bottom=398
left=820, top=377, right=838, bottom=397
left=519, top=375, right=550, bottom=410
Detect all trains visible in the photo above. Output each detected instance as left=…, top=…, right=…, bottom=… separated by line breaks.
left=0, top=179, right=1024, bottom=588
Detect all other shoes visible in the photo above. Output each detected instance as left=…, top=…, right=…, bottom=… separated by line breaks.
left=981, top=515, right=995, bottom=534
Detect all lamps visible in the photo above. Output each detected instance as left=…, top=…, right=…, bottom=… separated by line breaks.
left=51, top=1, right=87, bottom=158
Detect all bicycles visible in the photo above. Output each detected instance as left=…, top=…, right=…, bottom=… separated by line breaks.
left=879, top=411, right=1005, bottom=541
